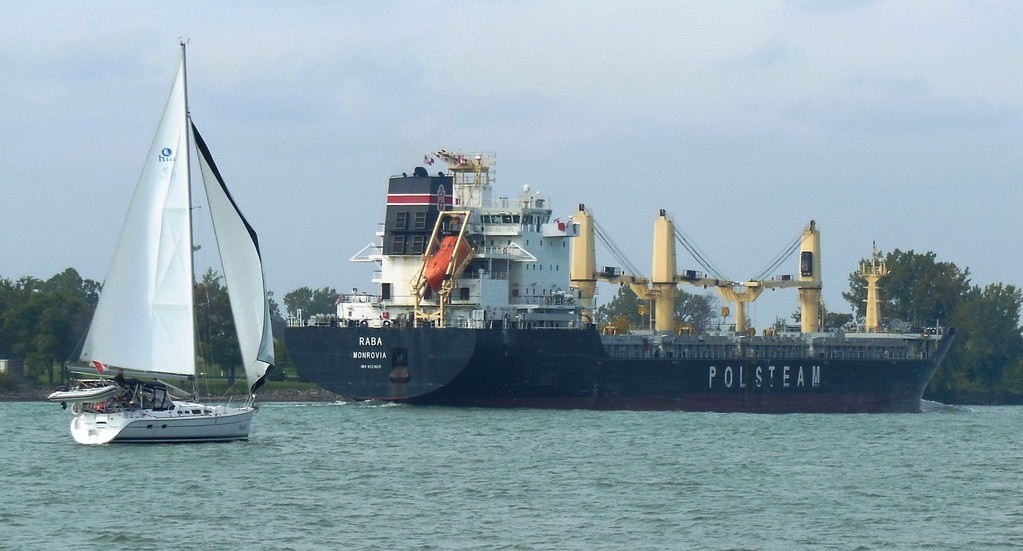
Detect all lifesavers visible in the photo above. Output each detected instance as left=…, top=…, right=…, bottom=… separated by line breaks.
left=382, top=320, right=390, bottom=327
left=503, top=248, right=507, bottom=255
left=359, top=320, right=368, bottom=327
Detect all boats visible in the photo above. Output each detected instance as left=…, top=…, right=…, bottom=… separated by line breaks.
left=287, top=149, right=955, bottom=412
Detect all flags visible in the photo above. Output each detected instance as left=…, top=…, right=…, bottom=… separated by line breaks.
left=424, top=156, right=434, bottom=166
left=94, top=360, right=109, bottom=372
left=458, top=156, right=467, bottom=164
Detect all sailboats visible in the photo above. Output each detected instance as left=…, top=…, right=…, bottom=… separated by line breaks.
left=70, top=35, right=277, bottom=446
